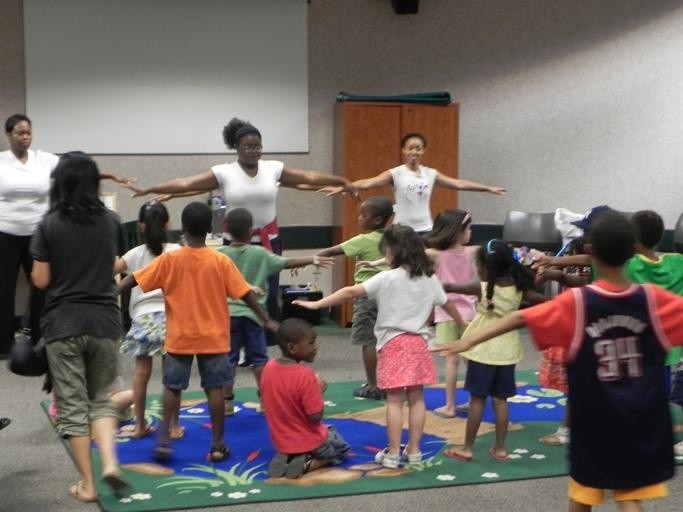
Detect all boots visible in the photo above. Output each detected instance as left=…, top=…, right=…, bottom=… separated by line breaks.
left=539, top=426, right=571, bottom=446
left=224, top=394, right=234, bottom=416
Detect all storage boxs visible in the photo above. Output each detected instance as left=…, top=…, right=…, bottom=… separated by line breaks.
left=273, top=282, right=324, bottom=327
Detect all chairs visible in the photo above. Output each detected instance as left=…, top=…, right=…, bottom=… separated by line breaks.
left=484, top=211, right=562, bottom=304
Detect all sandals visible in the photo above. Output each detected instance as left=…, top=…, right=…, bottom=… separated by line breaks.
left=211, top=444, right=230, bottom=462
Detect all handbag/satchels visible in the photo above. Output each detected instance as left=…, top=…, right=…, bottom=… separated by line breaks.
left=9, top=333, right=48, bottom=376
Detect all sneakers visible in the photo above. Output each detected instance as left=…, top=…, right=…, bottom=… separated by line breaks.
left=268, top=454, right=289, bottom=477
left=354, top=384, right=381, bottom=400
left=285, top=453, right=312, bottom=478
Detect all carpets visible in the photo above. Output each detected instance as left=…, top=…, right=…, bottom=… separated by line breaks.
left=29, top=365, right=678, bottom=512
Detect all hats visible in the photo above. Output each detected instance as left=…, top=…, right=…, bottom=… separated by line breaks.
left=571, top=206, right=612, bottom=231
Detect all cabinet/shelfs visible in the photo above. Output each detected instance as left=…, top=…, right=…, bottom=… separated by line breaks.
left=330, top=95, right=461, bottom=327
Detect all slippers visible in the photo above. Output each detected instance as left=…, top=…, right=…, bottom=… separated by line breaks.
left=69, top=481, right=99, bottom=503
left=133, top=425, right=156, bottom=439
left=375, top=447, right=400, bottom=469
left=455, top=405, right=469, bottom=413
left=444, top=449, right=472, bottom=461
left=490, top=447, right=507, bottom=461
left=433, top=408, right=452, bottom=418
left=170, top=426, right=185, bottom=438
left=402, top=445, right=422, bottom=465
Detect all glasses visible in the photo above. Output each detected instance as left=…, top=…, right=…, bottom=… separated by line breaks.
left=238, top=144, right=262, bottom=152
